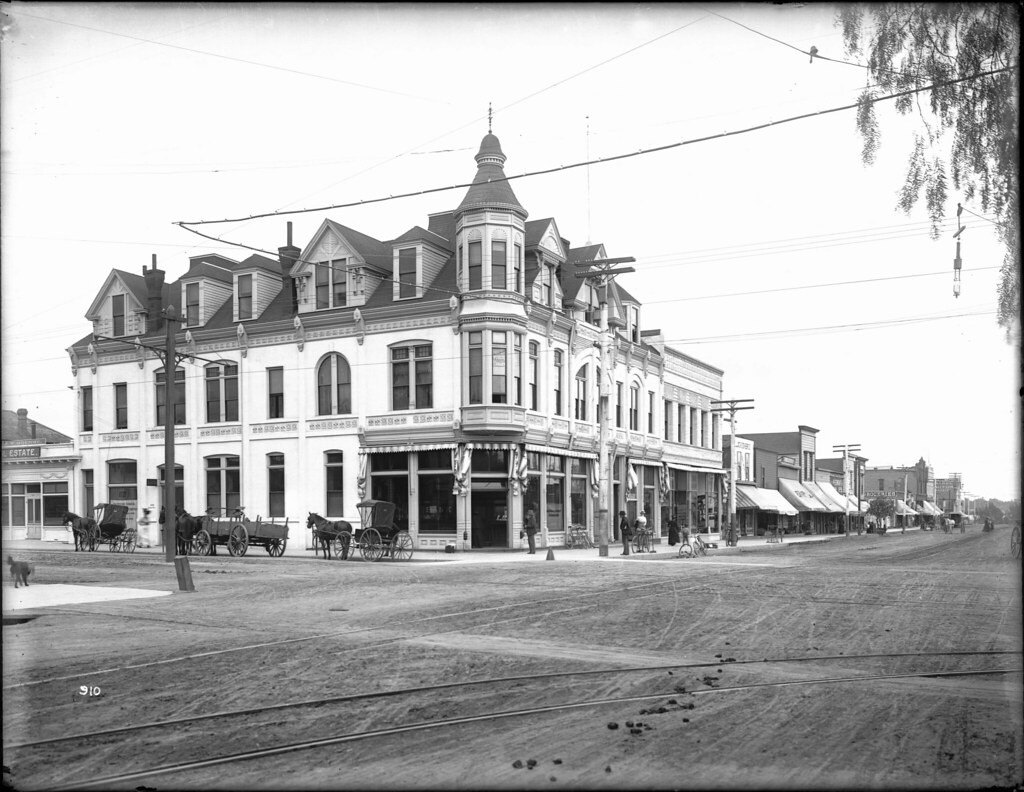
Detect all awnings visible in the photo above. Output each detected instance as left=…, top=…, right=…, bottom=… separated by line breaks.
left=734, top=477, right=945, bottom=516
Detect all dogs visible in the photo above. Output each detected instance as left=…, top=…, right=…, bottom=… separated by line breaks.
left=7, top=556, right=31, bottom=589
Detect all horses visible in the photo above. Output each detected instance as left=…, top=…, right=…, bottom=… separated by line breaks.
left=307, top=512, right=352, bottom=560
left=174, top=504, right=217, bottom=556
left=62, top=510, right=97, bottom=552
left=940, top=517, right=955, bottom=534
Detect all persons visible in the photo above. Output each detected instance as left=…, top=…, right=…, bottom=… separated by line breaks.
left=920, top=515, right=994, bottom=532
left=524, top=510, right=538, bottom=554
left=682, top=524, right=690, bottom=546
left=634, top=510, right=648, bottom=553
left=667, top=515, right=681, bottom=546
left=620, top=511, right=633, bottom=555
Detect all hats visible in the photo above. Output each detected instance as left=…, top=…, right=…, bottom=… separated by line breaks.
left=618, top=511, right=626, bottom=515
left=640, top=510, right=646, bottom=514
